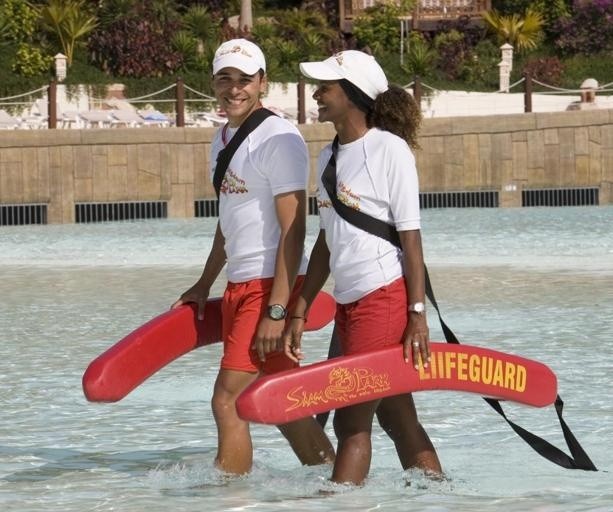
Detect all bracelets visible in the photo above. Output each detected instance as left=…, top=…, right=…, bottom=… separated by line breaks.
left=290, top=316, right=307, bottom=323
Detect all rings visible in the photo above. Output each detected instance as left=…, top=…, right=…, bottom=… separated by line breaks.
left=412, top=342, right=420, bottom=347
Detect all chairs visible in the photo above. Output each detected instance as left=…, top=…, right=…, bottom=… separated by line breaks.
left=0, top=98, right=229, bottom=130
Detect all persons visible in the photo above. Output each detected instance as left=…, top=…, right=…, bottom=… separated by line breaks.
left=273, top=49, right=449, bottom=498
left=169, top=37, right=335, bottom=475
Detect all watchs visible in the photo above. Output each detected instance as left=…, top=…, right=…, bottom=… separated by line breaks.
left=263, top=303, right=287, bottom=320
left=407, top=302, right=426, bottom=314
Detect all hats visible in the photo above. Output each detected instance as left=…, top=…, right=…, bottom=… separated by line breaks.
left=299, top=50, right=389, bottom=101
left=212, top=39, right=266, bottom=76
